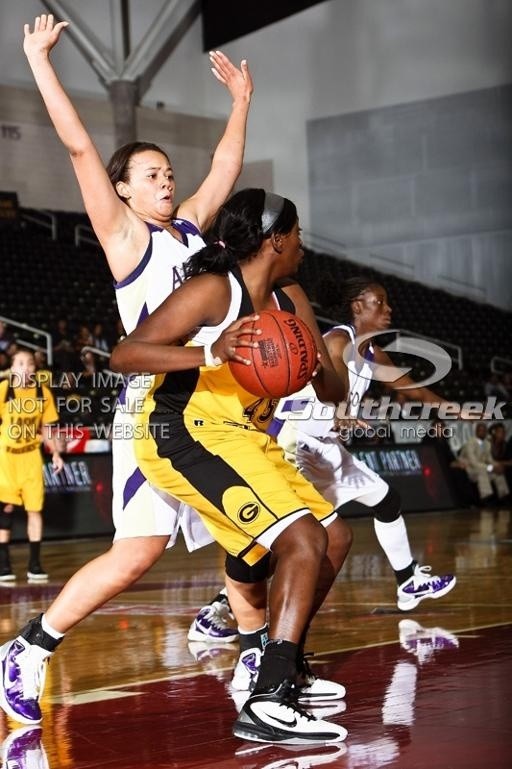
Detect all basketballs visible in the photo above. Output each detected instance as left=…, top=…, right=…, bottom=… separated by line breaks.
left=229, top=309, right=317, bottom=399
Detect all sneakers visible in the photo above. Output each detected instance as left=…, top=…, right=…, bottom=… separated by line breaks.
left=187, top=605, right=241, bottom=642
left=0, top=566, right=17, bottom=581
left=26, top=566, right=49, bottom=581
left=230, top=647, right=349, bottom=746
left=396, top=564, right=457, bottom=613
left=1, top=725, right=50, bottom=769
left=0, top=635, right=55, bottom=724
left=398, top=618, right=460, bottom=664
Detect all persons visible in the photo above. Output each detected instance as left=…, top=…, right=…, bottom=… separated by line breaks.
left=1, top=12, right=256, bottom=726
left=188, top=618, right=462, bottom=769
left=0, top=318, right=512, bottom=511
left=185, top=276, right=491, bottom=644
left=0, top=349, right=66, bottom=581
left=106, top=188, right=352, bottom=748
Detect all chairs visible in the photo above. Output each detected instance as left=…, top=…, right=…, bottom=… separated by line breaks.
left=0, top=202, right=512, bottom=445
